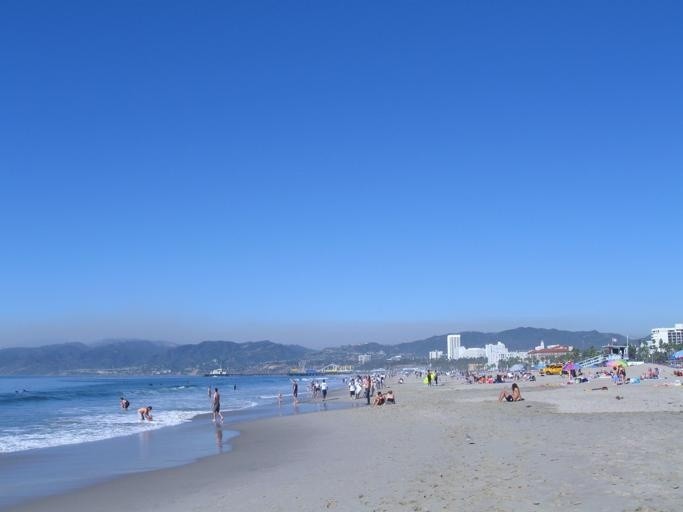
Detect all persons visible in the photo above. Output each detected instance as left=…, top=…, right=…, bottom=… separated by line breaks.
left=210, top=387, right=223, bottom=422
left=118, top=396, right=128, bottom=409
left=286, top=359, right=664, bottom=405
left=136, top=406, right=151, bottom=420
left=213, top=420, right=224, bottom=449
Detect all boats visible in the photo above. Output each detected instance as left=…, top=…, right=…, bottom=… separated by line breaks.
left=208, top=368, right=228, bottom=377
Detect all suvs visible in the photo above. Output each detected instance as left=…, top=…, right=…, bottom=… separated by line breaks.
left=538, top=363, right=564, bottom=376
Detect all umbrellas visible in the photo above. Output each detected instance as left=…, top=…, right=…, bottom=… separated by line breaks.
left=673, top=349, right=683, bottom=359
